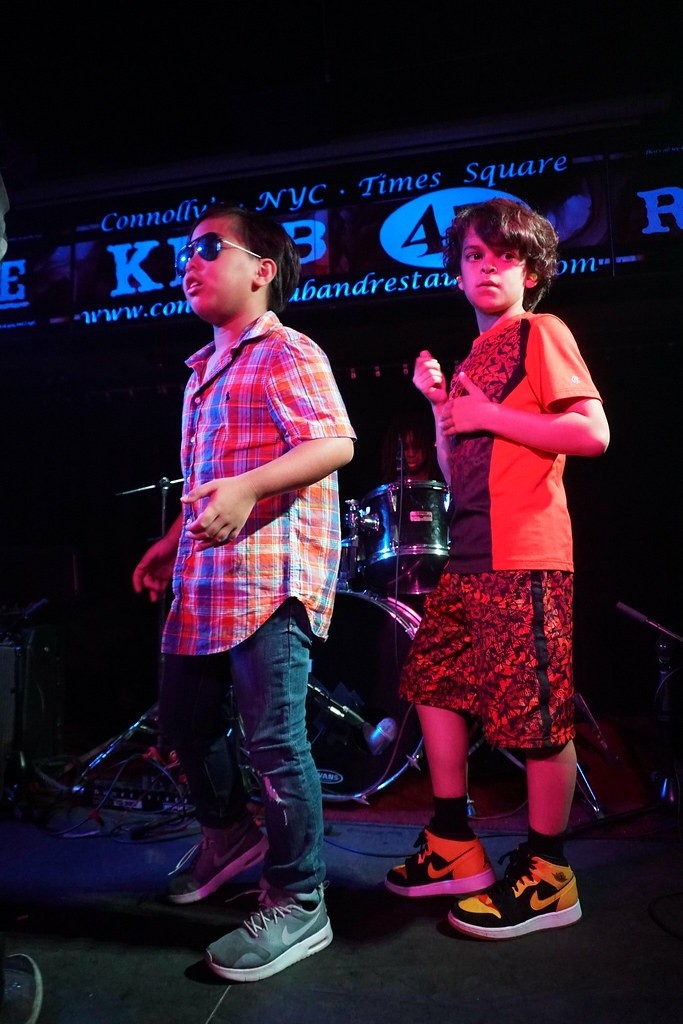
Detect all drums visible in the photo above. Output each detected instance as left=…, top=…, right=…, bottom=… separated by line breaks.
left=361, top=481, right=448, bottom=594
left=304, top=588, right=422, bottom=806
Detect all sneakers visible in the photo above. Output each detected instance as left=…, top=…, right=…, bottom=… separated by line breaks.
left=448, top=840, right=584, bottom=940
left=383, top=826, right=496, bottom=898
left=206, top=878, right=334, bottom=983
left=167, top=816, right=270, bottom=905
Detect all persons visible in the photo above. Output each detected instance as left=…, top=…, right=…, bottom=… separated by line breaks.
left=388, top=420, right=447, bottom=486
left=133, top=206, right=358, bottom=982
left=0, top=952, right=43, bottom=1024
left=385, top=197, right=609, bottom=938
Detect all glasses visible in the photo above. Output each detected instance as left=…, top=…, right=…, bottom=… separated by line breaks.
left=173, top=230, right=263, bottom=278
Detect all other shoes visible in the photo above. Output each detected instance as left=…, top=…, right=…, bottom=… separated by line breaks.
left=0, top=953, right=43, bottom=1024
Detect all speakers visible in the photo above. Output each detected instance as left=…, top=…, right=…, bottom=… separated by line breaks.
left=0, top=621, right=66, bottom=757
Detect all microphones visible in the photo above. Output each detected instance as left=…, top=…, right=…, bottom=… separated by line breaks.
left=367, top=717, right=400, bottom=756
